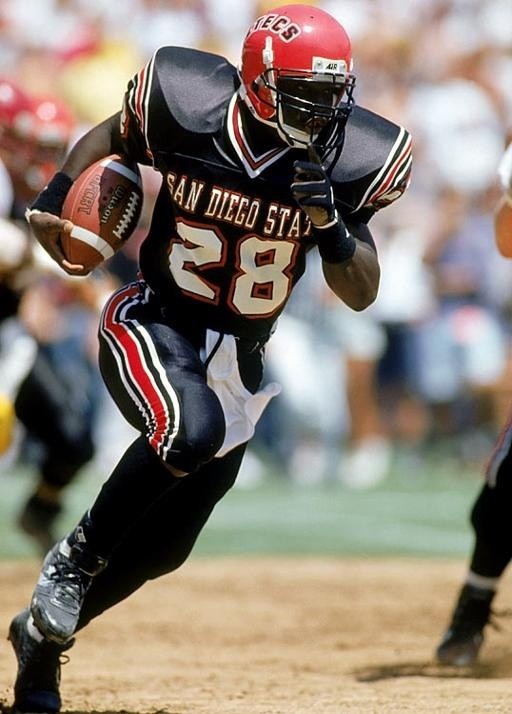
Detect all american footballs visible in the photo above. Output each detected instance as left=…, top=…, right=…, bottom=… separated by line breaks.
left=60, top=154, right=143, bottom=273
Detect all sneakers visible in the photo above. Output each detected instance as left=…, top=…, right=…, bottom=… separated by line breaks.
left=30, top=522, right=95, bottom=647
left=7, top=608, right=63, bottom=712
left=436, top=579, right=495, bottom=667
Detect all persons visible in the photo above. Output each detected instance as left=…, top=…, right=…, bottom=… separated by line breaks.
left=8, top=4, right=410, bottom=711
left=426, top=416, right=510, bottom=670
left=1, top=1, right=511, bottom=557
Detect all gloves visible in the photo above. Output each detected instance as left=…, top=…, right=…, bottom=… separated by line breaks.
left=290, top=145, right=355, bottom=266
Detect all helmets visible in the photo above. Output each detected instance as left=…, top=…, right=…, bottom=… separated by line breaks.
left=240, top=3, right=354, bottom=120
